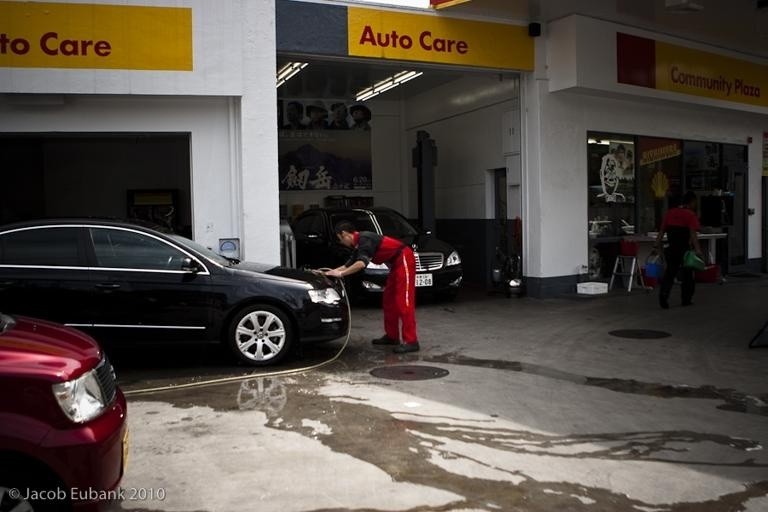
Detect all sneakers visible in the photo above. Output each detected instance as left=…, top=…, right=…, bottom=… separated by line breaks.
left=371, top=335, right=401, bottom=346
left=658, top=293, right=694, bottom=309
left=391, top=341, right=420, bottom=353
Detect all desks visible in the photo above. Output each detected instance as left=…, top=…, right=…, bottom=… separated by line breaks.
left=639, top=231, right=729, bottom=264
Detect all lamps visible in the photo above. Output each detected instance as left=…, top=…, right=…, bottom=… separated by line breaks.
left=277, top=61, right=308, bottom=92
left=355, top=71, right=424, bottom=103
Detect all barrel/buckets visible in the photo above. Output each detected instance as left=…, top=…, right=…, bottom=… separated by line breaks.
left=644, top=252, right=665, bottom=277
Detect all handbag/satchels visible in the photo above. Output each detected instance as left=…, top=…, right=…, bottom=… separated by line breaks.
left=645, top=248, right=674, bottom=277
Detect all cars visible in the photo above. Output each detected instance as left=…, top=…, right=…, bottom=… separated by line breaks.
left=0, top=309, right=129, bottom=512
left=290, top=204, right=465, bottom=301
left=588, top=212, right=613, bottom=279
left=0, top=216, right=350, bottom=366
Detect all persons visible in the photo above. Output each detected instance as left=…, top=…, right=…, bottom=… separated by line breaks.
left=310, top=220, right=420, bottom=353
left=611, top=143, right=634, bottom=176
left=304, top=101, right=329, bottom=129
left=348, top=104, right=372, bottom=130
left=282, top=100, right=307, bottom=130
left=650, top=189, right=704, bottom=310
left=327, top=102, right=350, bottom=131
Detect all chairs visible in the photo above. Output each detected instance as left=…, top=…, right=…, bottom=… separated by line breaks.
left=609, top=239, right=654, bottom=296
